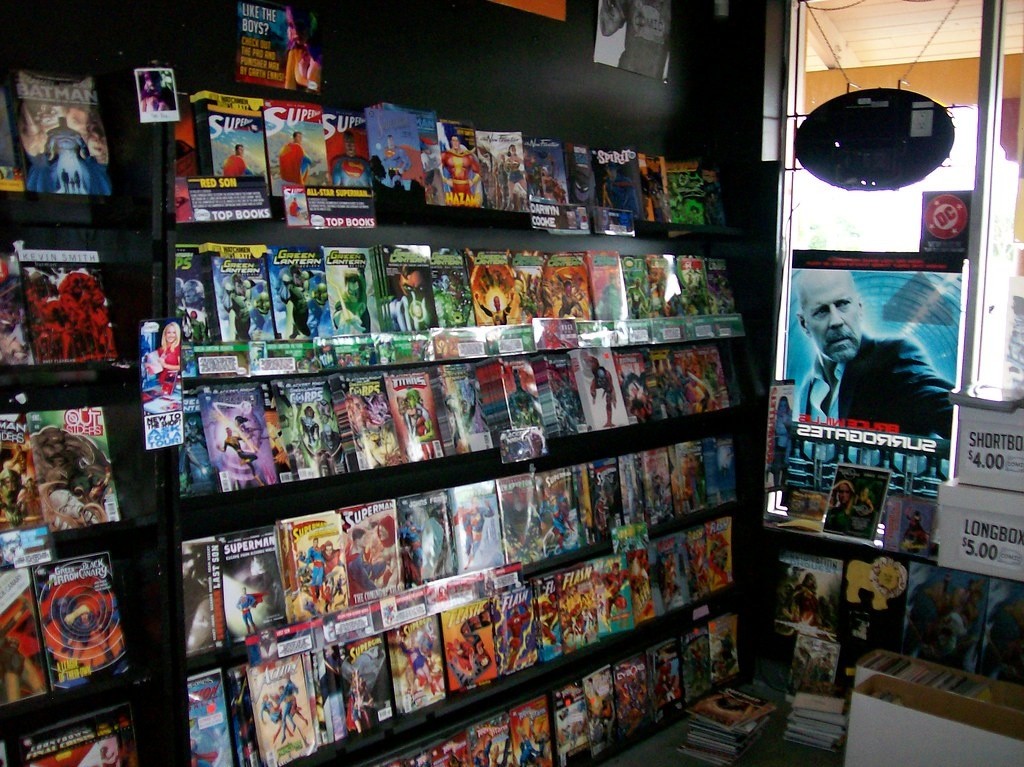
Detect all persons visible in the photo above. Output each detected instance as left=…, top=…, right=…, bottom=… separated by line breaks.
left=771, top=396, right=793, bottom=487
left=280, top=132, right=313, bottom=185
left=222, top=144, right=255, bottom=176
left=795, top=268, right=955, bottom=440
left=143, top=321, right=180, bottom=395
left=283, top=2, right=323, bottom=96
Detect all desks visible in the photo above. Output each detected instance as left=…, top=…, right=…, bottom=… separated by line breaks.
left=843, top=691, right=1024, bottom=767
left=761, top=521, right=936, bottom=566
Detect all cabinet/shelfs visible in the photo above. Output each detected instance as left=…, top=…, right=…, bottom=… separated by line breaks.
left=0, top=51, right=756, bottom=767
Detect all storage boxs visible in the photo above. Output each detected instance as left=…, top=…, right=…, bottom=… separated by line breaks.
left=937, top=476, right=1024, bottom=582
left=855, top=649, right=1023, bottom=743
left=955, top=405, right=1024, bottom=493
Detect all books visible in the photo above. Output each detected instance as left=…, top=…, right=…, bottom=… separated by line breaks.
left=0, top=1, right=1024, bottom=767
